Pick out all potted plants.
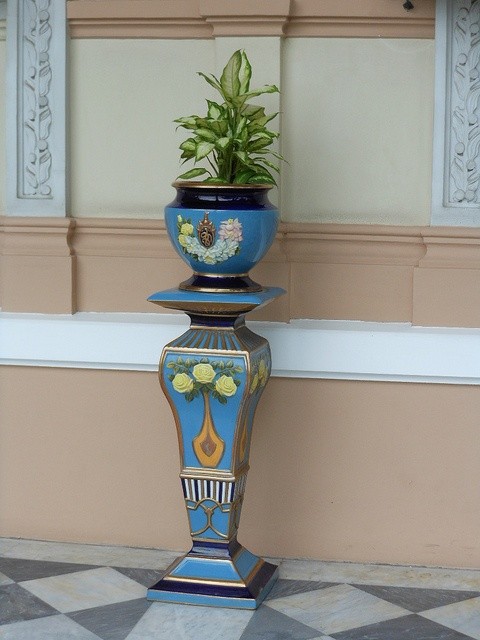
[166,50,288,294]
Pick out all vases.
[146,291,279,609]
